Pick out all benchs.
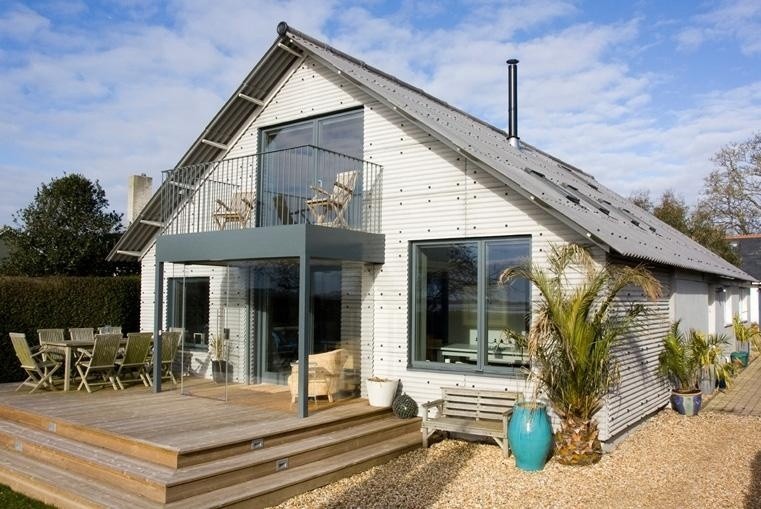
[421,386,528,458]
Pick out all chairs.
[10,322,182,396]
[306,169,358,229]
[214,191,255,231]
[272,196,313,225]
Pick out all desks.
[441,341,531,364]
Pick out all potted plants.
[730,316,761,370]
[208,335,232,384]
[656,318,742,417]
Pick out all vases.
[508,399,552,470]
[367,376,401,408]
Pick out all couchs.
[286,347,349,403]
